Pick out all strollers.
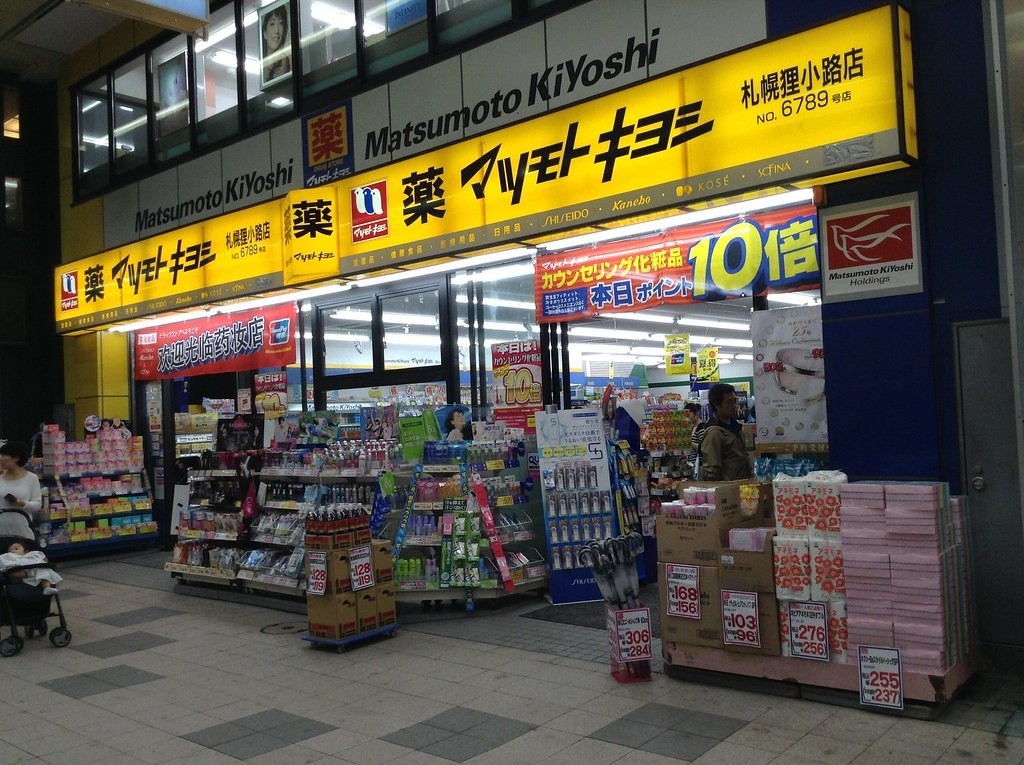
[0,509,72,657]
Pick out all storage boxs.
[656,479,976,674]
[303,519,397,640]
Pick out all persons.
[446,409,465,442]
[362,408,380,438]
[8,543,58,595]
[606,399,614,419]
[685,403,706,467]
[0,441,42,541]
[276,417,336,439]
[701,384,752,482]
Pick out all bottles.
[252,440,395,533]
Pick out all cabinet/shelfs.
[34,468,160,560]
[163,456,548,614]
